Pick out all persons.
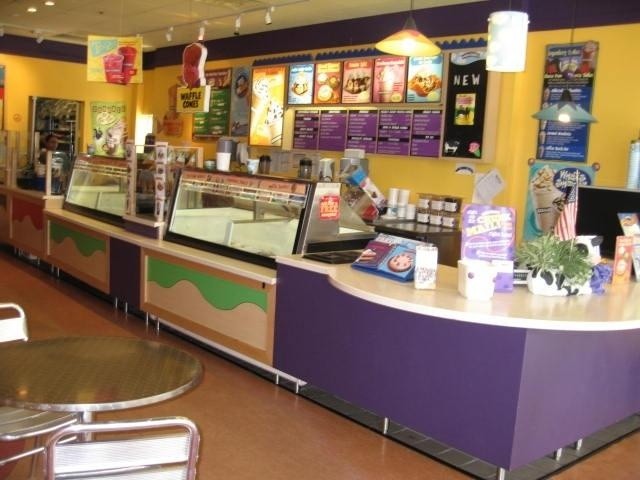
[38,134,59,165]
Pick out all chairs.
[42,415,202,480]
[0,302,83,479]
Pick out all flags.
[553,178,579,246]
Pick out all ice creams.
[529,165,557,231]
[250,77,284,146]
[467,141,481,157]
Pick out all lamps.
[483,2,531,76]
[0,25,45,44]
[162,5,273,44]
[531,1,597,128]
[373,0,442,60]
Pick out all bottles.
[298,159,312,179]
[416,191,462,228]
[259,155,271,174]
[627,139,640,190]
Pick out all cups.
[414,246,439,290]
[104,46,138,79]
[387,188,417,220]
[246,159,260,174]
[531,189,562,232]
[205,160,214,171]
[215,152,232,171]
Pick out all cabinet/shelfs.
[366,215,464,269]
[164,163,377,272]
[61,153,177,246]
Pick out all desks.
[0,335,207,443]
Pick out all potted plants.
[516,233,593,299]
